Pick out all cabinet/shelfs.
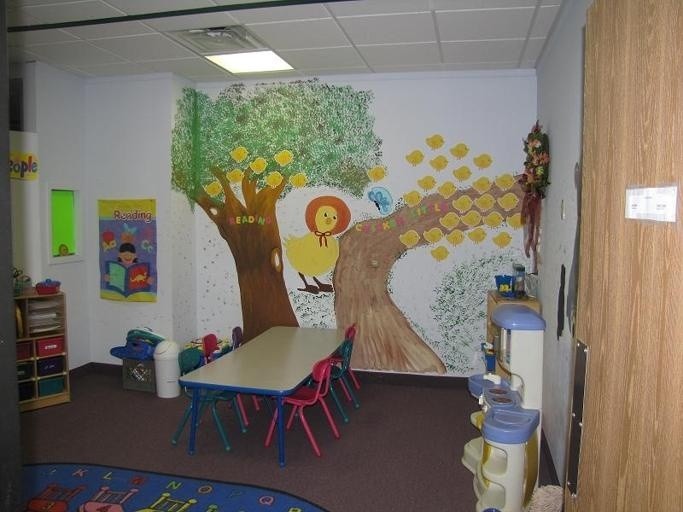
[12,285,71,413]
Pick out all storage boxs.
[16,335,65,402]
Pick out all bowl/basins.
[36,282,62,295]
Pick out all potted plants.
[11,265,31,296]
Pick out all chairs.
[171,322,361,468]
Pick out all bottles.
[46,279,52,287]
[514,264,525,291]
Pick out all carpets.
[20,463,328,512]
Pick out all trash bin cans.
[152,341,182,399]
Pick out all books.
[104,260,150,297]
[27,298,64,336]
